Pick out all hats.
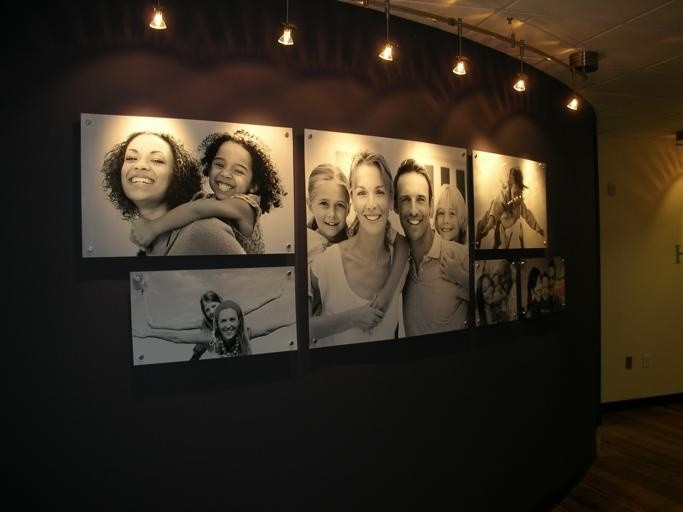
[508,168,529,189]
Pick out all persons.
[475,261,518,326]
[100,131,285,257]
[132,300,296,358]
[147,291,285,329]
[526,262,563,317]
[307,151,470,348]
[476,167,545,250]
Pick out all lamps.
[149,0,167,30]
[512,41,526,92]
[452,19,467,76]
[566,66,580,111]
[379,3,394,62]
[278,1,295,46]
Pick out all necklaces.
[218,336,239,356]
